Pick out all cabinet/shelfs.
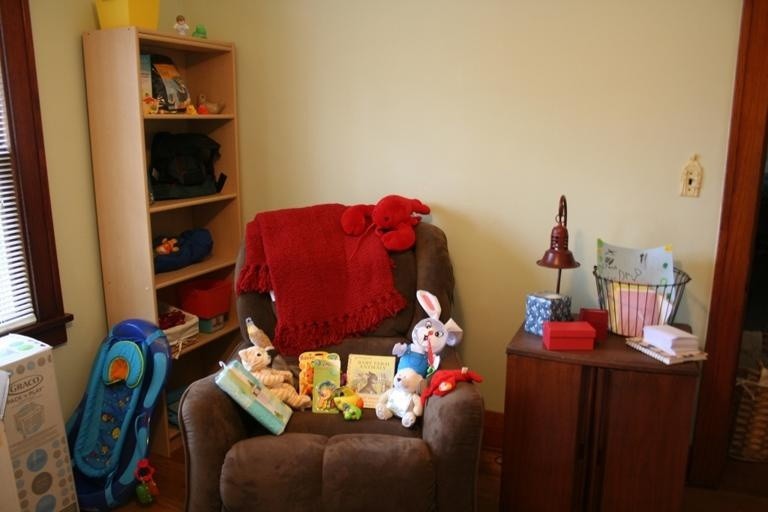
[500,323,700,511]
[82,27,245,457]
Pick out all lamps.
[536,194,579,294]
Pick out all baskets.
[591,267,691,337]
[159,300,199,355]
[728,364,767,465]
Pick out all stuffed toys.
[375,364,424,430]
[392,290,464,375]
[156,237,179,255]
[239,344,311,411]
[339,192,433,252]
[244,314,301,394]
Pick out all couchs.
[176,204,485,512]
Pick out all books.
[295,350,398,421]
[626,332,709,366]
